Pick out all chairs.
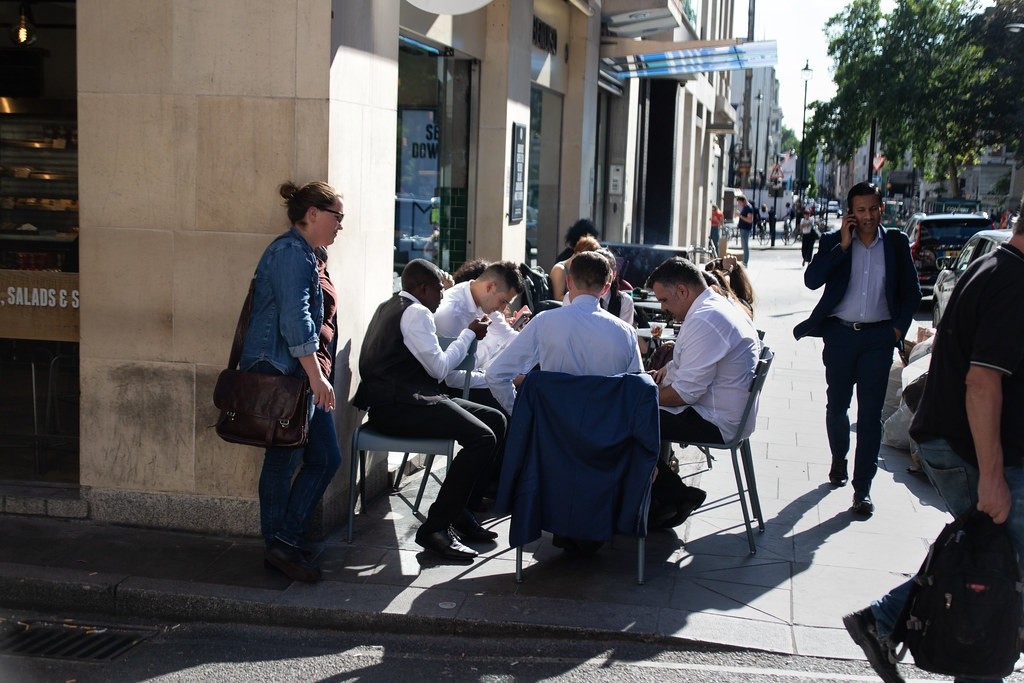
[347,264,775,585]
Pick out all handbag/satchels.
[213,368,309,447]
[812,228,821,240]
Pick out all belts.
[833,316,891,330]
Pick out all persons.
[237,180,343,584]
[842,190,1024,683]
[793,180,921,513]
[703,203,815,269]
[736,195,753,268]
[651,255,760,529]
[485,250,645,556]
[352,257,507,558]
[430,216,758,499]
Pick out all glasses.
[319,206,344,222]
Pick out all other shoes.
[676,485,705,516]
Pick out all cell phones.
[848,210,854,235]
[512,310,532,330]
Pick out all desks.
[601,241,689,284]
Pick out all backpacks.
[911,507,1024,674]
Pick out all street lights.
[752,89,765,240]
[796,58,814,225]
[769,152,786,248]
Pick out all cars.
[881,200,904,228]
[793,195,839,216]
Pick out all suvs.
[931,228,1015,331]
[902,209,998,310]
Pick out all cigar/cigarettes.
[328,402,336,413]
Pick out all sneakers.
[457,513,497,541]
[829,457,849,487]
[852,493,874,514]
[844,608,906,683]
[264,541,322,583]
[415,519,480,560]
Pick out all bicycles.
[781,217,797,246]
[756,219,771,246]
[722,218,731,241]
